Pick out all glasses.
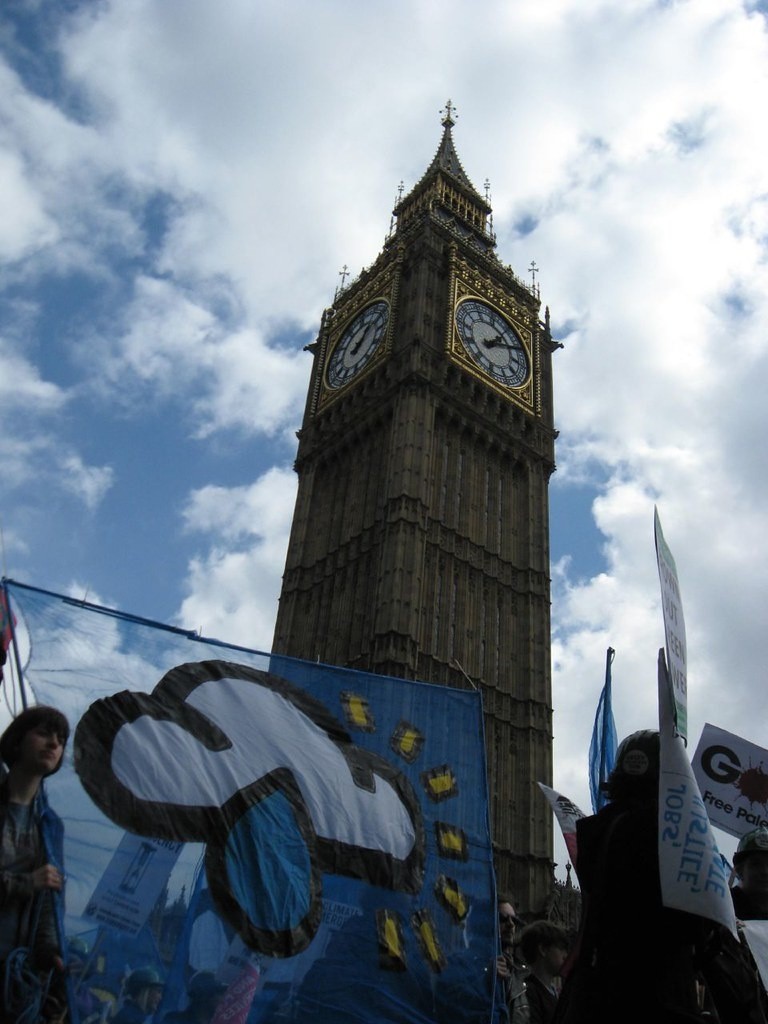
[498,912,520,925]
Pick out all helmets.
[732,825,768,875]
[608,729,660,797]
[67,938,87,955]
[121,966,167,996]
[185,969,228,998]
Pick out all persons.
[67,935,230,1024]
[498,730,768,1024]
[0,705,71,1024]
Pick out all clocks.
[322,297,391,391]
[453,296,532,390]
